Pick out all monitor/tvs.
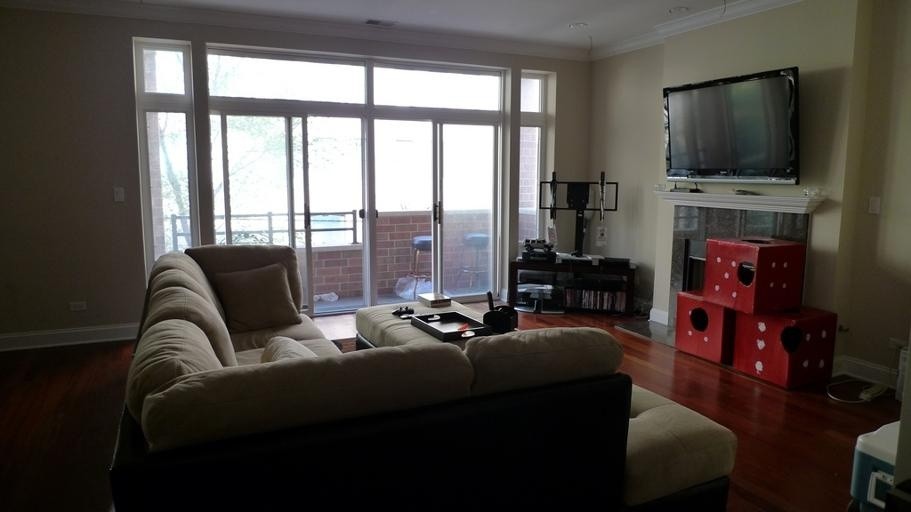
[663,66,800,184]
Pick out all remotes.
[399,313,424,319]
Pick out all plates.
[411,310,493,343]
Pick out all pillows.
[261,336,319,363]
[216,262,302,332]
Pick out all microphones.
[550,171,558,219]
[599,172,606,220]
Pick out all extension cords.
[859,383,890,403]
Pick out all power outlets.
[887,337,908,351]
[69,301,87,311]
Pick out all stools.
[408,236,432,301]
[454,233,490,290]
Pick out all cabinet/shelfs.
[509,262,634,316]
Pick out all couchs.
[110,245,739,512]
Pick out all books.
[417,292,451,307]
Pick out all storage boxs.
[851,420,901,509]
